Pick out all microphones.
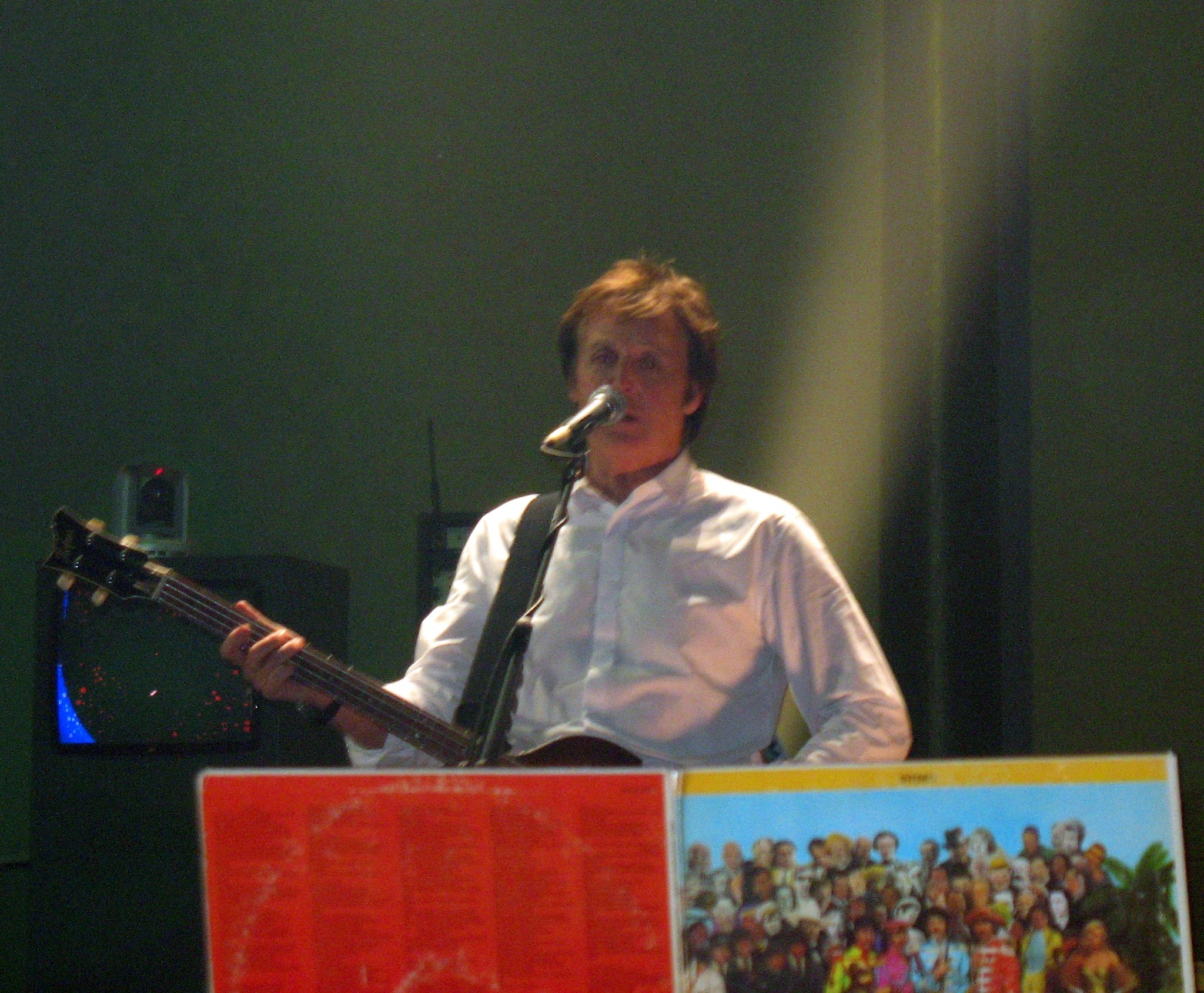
[540,384,627,451]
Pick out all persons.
[682,819,1139,993]
[222,258,911,770]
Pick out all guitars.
[37,501,658,768]
